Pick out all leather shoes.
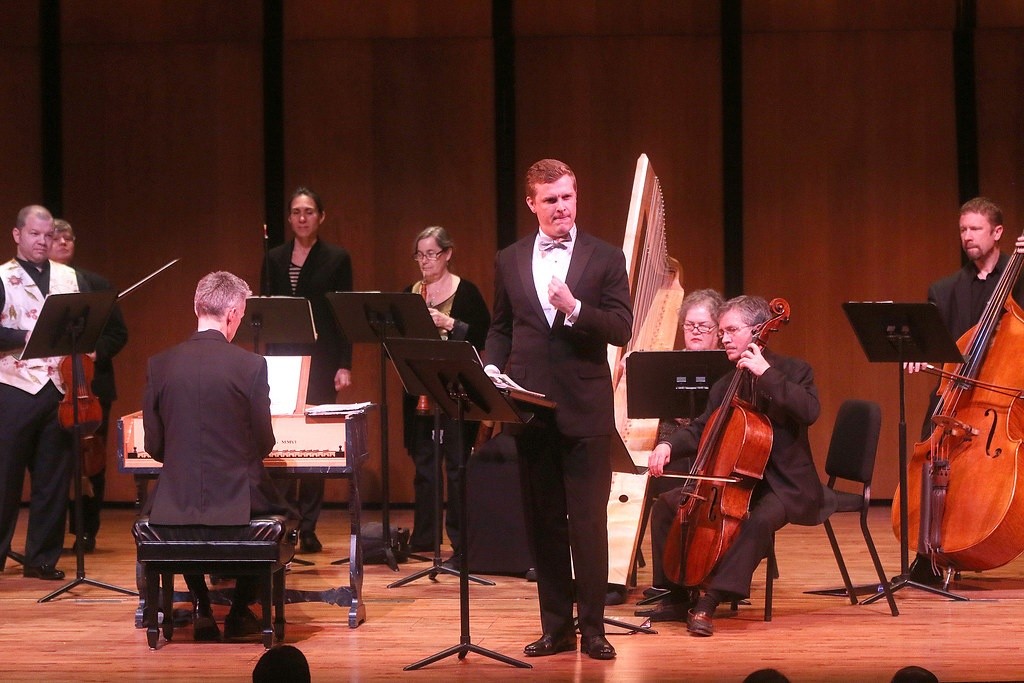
[581,634,618,660]
[634,598,691,622]
[192,599,220,639]
[524,634,577,656]
[739,600,751,605]
[224,613,276,636]
[73,533,95,553]
[23,564,65,578]
[687,608,713,635]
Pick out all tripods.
[842,301,970,605]
[626,349,753,608]
[326,290,536,672]
[20,289,140,604]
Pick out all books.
[490,371,545,398]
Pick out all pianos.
[116,402,375,630]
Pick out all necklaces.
[427,277,446,306]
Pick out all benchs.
[131,514,301,653]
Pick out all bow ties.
[538,231,572,251]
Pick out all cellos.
[890,250,1023,592]
[661,297,793,588]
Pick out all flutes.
[417,271,430,411]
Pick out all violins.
[57,354,104,441]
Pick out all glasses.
[681,320,718,333]
[412,249,444,262]
[53,234,76,245]
[716,322,762,338]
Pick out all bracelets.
[659,442,672,448]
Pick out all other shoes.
[440,555,460,574]
[299,533,323,552]
[406,542,436,553]
[891,563,944,586]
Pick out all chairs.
[731,399,899,623]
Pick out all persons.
[0,205,97,580]
[260,186,354,551]
[903,197,1024,584]
[891,666,939,683]
[387,227,491,571]
[50,219,130,553]
[253,645,311,683]
[482,157,633,660]
[643,287,836,635]
[141,271,302,641]
[743,669,789,683]
